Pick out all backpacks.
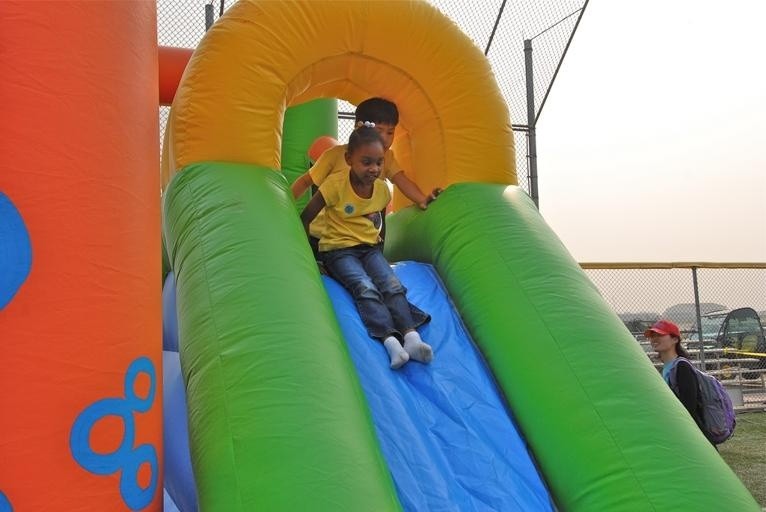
[669,357,736,444]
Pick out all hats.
[644,320,680,337]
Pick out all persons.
[291,97,444,263]
[644,321,720,453]
[300,126,433,370]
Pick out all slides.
[162,162,762,512]
